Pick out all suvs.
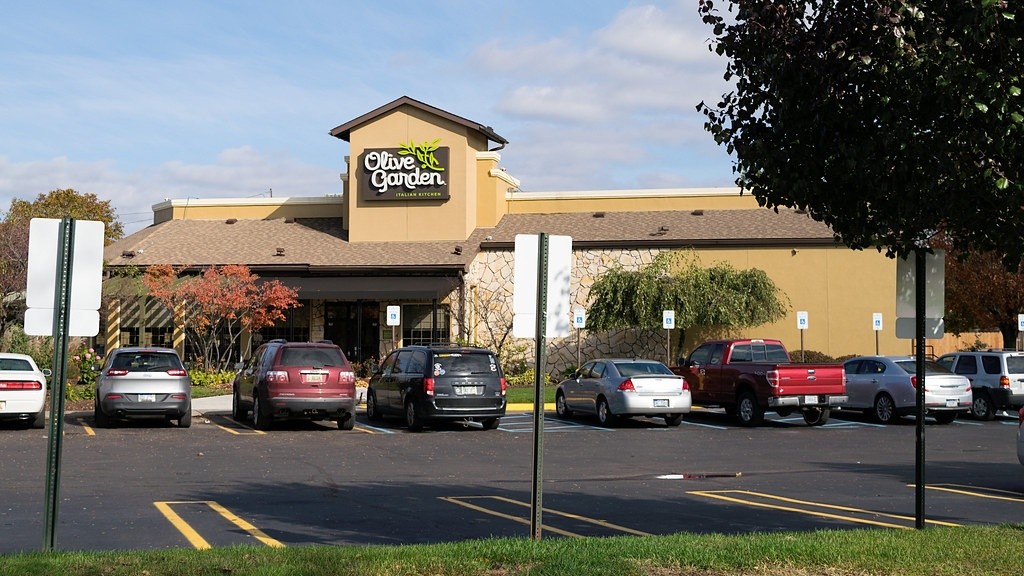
[932,350,1024,419]
[91,348,194,429]
[363,344,506,429]
[229,337,358,431]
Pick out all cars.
[553,355,695,427]
[0,351,53,428]
[826,355,974,429]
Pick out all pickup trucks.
[665,337,850,428]
